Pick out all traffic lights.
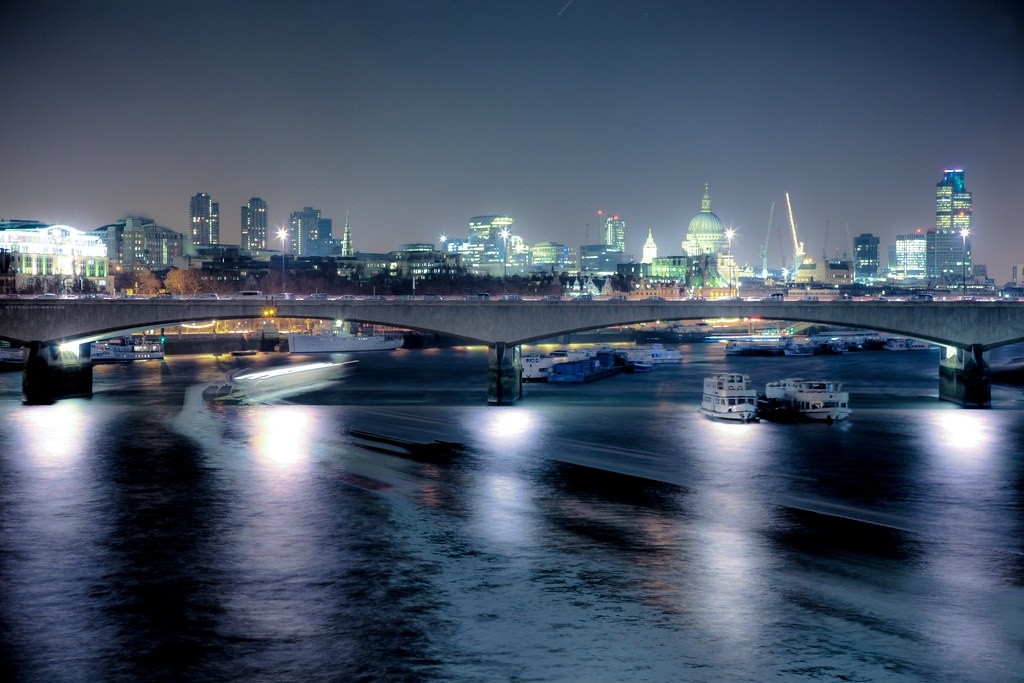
[161,335,165,344]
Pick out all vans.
[229,290,262,298]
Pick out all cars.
[541,292,851,301]
[271,293,524,301]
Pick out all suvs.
[200,294,219,301]
[906,293,933,303]
[149,291,174,300]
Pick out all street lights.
[279,228,287,291]
[726,230,733,299]
[501,229,508,292]
[961,228,968,293]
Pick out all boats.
[520,342,683,384]
[698,371,757,423]
[86,336,165,361]
[724,340,849,357]
[283,328,405,354]
[702,339,721,343]
[1,347,25,369]
[884,336,929,351]
[632,323,714,345]
[756,376,852,422]
[234,350,255,355]
